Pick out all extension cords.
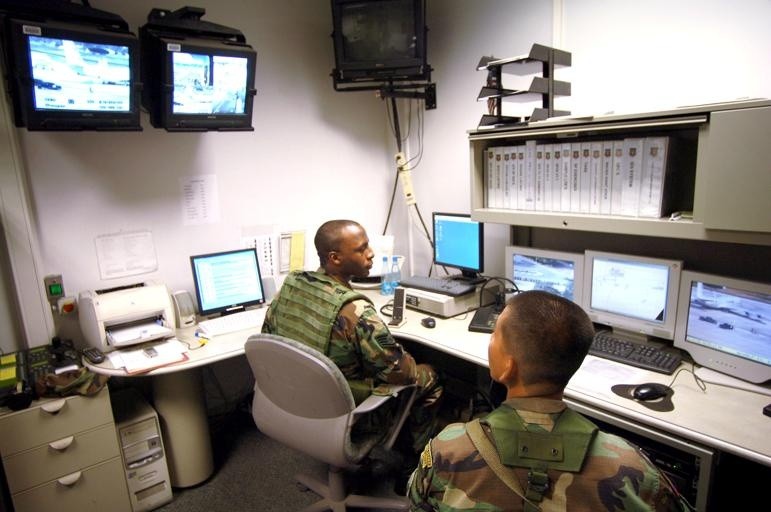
[394,151,416,205]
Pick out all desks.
[76,284,771,510]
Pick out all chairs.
[245,332,418,512]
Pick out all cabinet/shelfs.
[476,44,572,128]
[0,380,131,512]
[467,103,706,240]
[700,99,770,246]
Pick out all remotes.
[82,347,104,364]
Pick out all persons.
[255,217,448,495]
[405,287,698,512]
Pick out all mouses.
[634,383,673,401]
[422,318,436,329]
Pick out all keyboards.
[586,329,682,372]
[198,306,269,338]
[469,309,500,333]
[400,275,476,297]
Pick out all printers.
[78,272,176,354]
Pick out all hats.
[36,367,109,397]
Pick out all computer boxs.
[111,386,174,512]
[396,285,500,317]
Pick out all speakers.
[172,289,197,329]
[262,276,277,304]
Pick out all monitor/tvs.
[332,0,426,80]
[674,268,771,384]
[140,7,256,132]
[504,255,582,304]
[0,0,140,131]
[433,212,487,285]
[585,249,682,347]
[190,248,266,316]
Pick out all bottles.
[391,256,401,289]
[382,256,391,295]
